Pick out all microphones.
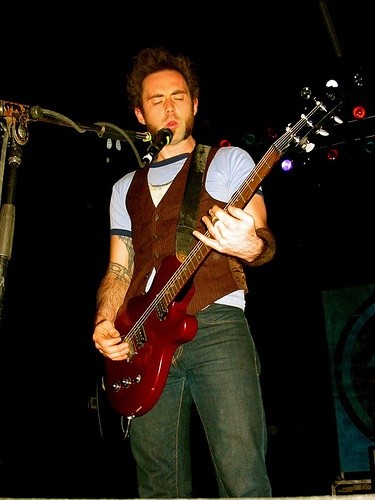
[140,127,174,168]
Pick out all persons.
[90,54,277,500]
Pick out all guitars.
[102,84,344,440]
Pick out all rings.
[212,218,220,227]
[102,352,108,356]
